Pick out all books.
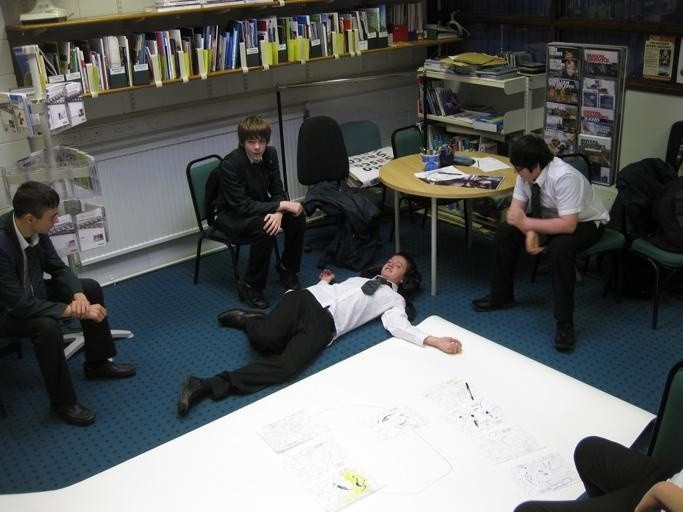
[12,2,682,98]
[418,99,622,221]
[344,144,395,190]
[0,81,111,255]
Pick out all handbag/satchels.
[204,166,218,227]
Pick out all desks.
[378,151,519,296]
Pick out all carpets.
[1,202,683,494]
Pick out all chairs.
[391,125,468,234]
[0,316,73,416]
[630,120,683,330]
[530,152,625,282]
[297,115,389,271]
[186,154,280,303]
[645,360,683,479]
[340,120,387,248]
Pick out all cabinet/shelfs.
[416,69,546,239]
[426,0,683,97]
[4,0,465,122]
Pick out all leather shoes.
[274,260,301,291]
[82,359,136,379]
[176,374,202,418]
[553,314,576,352]
[49,401,96,426]
[235,279,271,310]
[217,308,267,332]
[470,285,516,311]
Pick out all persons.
[204,115,307,308]
[473,134,610,350]
[0,181,136,427]
[176,250,461,415]
[513,436,682,511]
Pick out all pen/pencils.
[418,145,441,155]
[477,161,479,167]
[466,382,474,400]
[437,172,462,175]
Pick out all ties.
[530,183,541,218]
[24,245,49,301]
[360,275,393,296]
